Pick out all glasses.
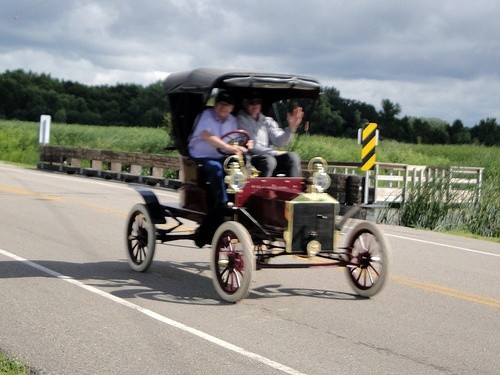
[248,100,263,104]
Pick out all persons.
[187,91,254,210]
[234,92,306,179]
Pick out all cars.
[125,66,389,302]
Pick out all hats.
[215,90,236,104]
